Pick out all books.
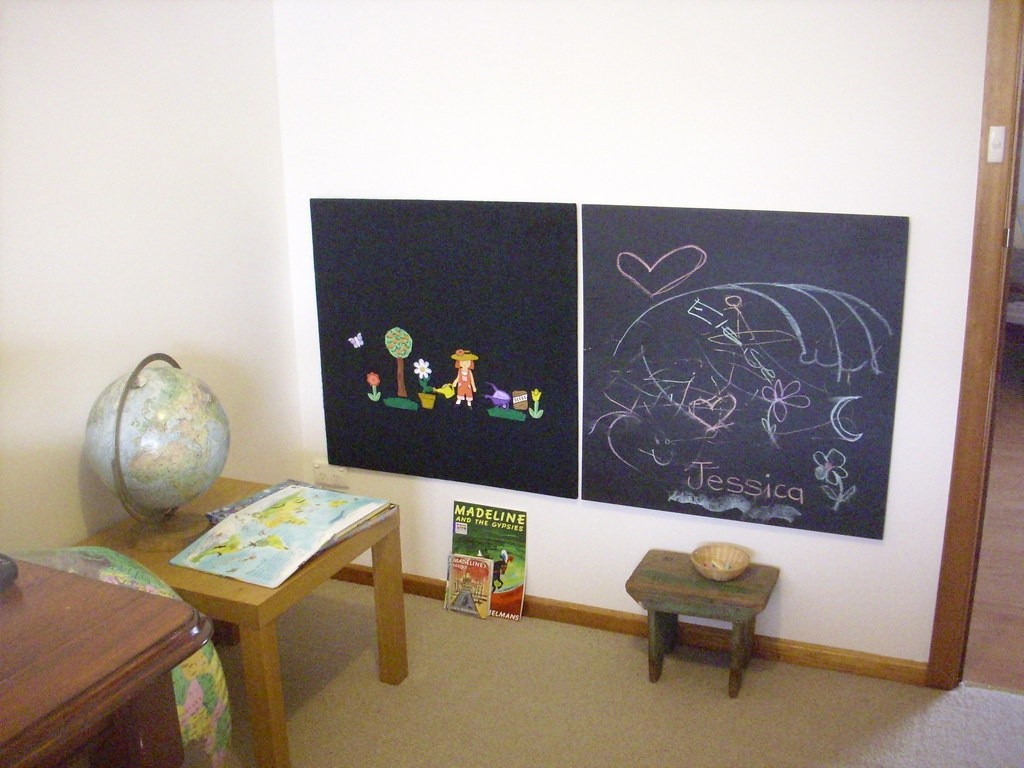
[168,475,395,588]
[442,499,528,621]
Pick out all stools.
[624,549,782,699]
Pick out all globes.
[1,545,231,767]
[85,352,230,553]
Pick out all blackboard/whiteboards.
[310,197,579,498]
[580,202,909,540]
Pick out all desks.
[71,470,410,768]
[1,552,216,768]
